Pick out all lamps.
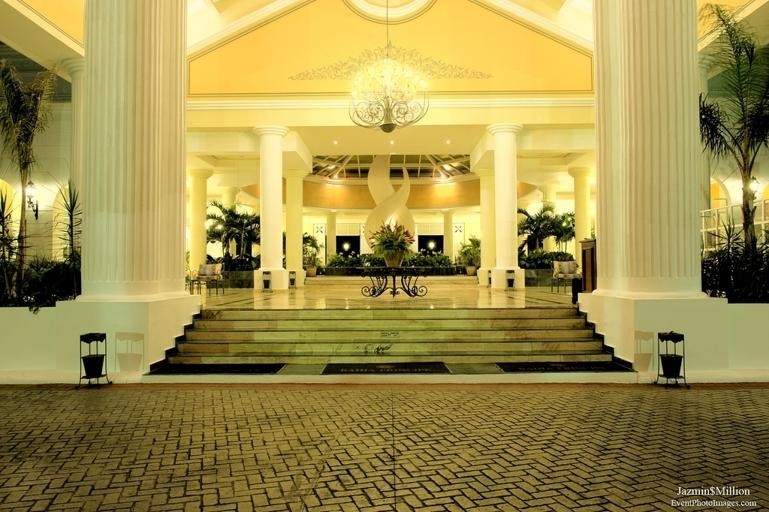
[348,1,430,133]
[26,180,39,220]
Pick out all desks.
[360,266,428,298]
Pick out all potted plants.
[303,248,322,277]
[458,235,481,276]
[368,217,419,267]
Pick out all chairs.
[550,260,581,294]
[190,263,226,295]
